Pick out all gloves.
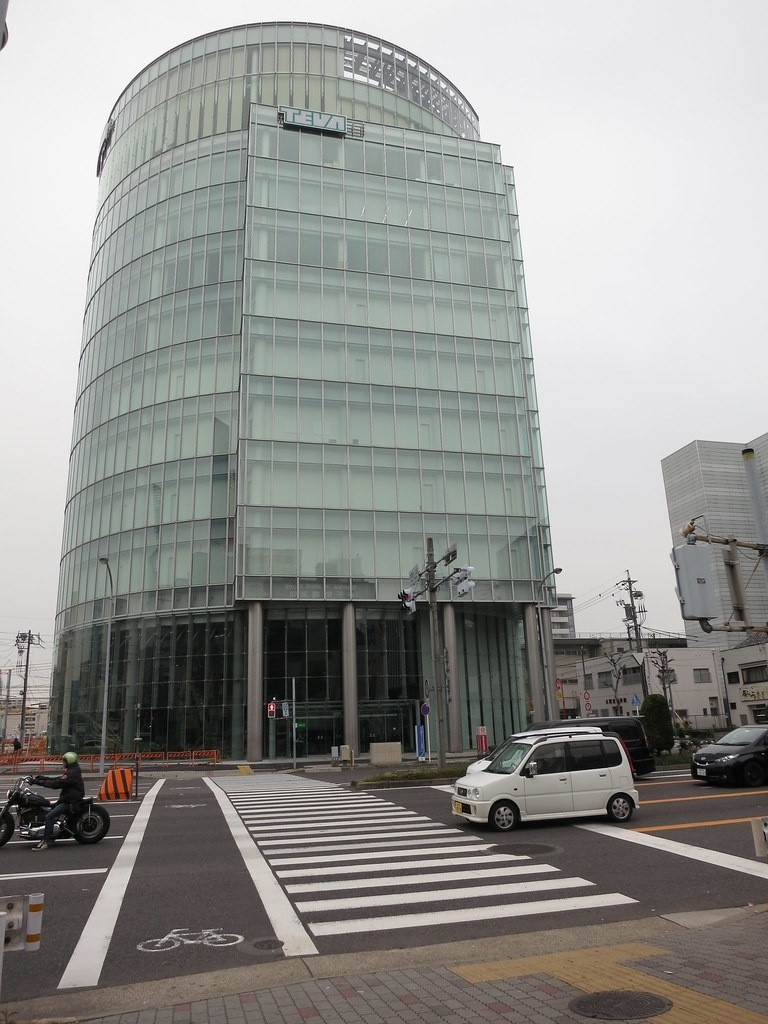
[35,775,49,780]
[29,779,44,786]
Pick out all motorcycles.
[0,774,111,847]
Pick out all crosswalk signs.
[631,695,641,707]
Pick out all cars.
[691,725,768,785]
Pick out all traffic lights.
[268,703,276,719]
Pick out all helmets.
[62,752,78,769]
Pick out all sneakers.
[32,840,48,852]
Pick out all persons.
[28,752,85,851]
[13,737,21,751]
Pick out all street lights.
[99,557,113,774]
[537,568,565,720]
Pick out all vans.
[522,716,654,777]
[450,733,639,830]
[466,726,602,776]
